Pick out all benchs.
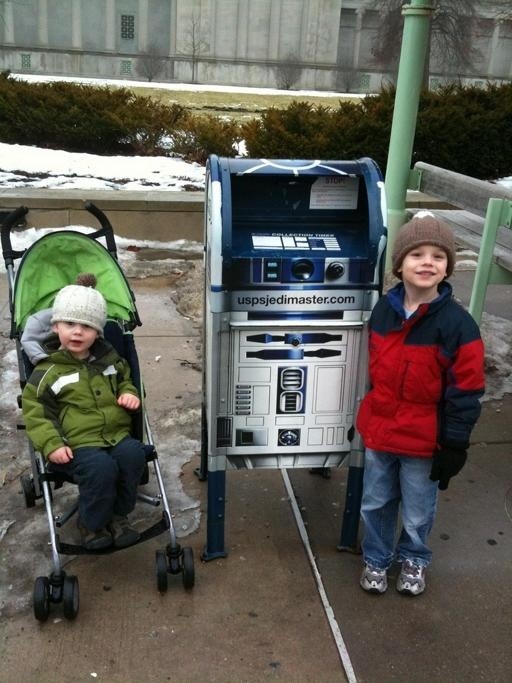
[413,162,511,327]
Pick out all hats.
[48,284,107,337]
[391,216,456,281]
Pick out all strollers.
[1,202,195,623]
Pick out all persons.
[22,271,148,551]
[356,208,485,597]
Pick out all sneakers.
[75,512,142,553]
[357,555,427,598]
